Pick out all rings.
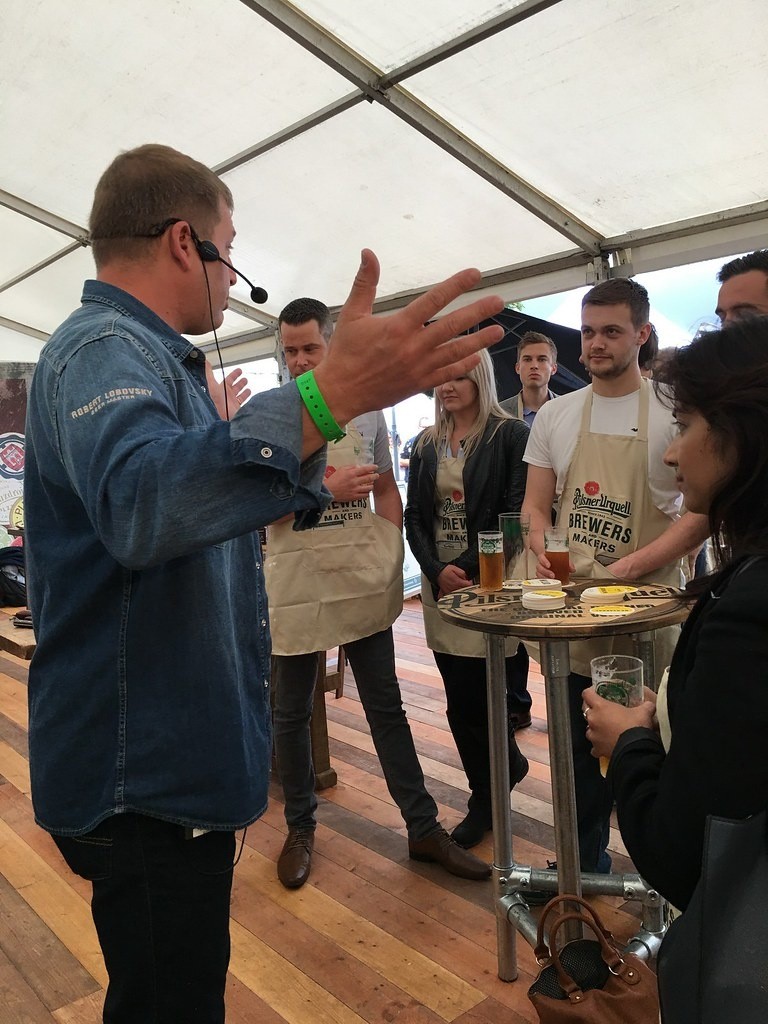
[583,708,591,719]
[585,725,590,731]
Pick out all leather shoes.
[408,829,494,880]
[277,826,314,890]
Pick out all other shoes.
[580,844,612,877]
[507,711,532,729]
[509,756,530,796]
[520,862,557,911]
[449,799,489,849]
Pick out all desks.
[0,603,342,789]
[437,576,697,983]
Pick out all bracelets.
[295,369,347,446]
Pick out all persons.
[638,320,711,580]
[404,337,533,850]
[497,330,563,726]
[714,249,768,328]
[580,313,768,914]
[519,277,715,906]
[25,145,503,1024]
[259,298,492,891]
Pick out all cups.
[498,512,530,586]
[478,531,504,592]
[590,655,644,777]
[544,526,569,585]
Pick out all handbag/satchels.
[527,894,660,1024]
